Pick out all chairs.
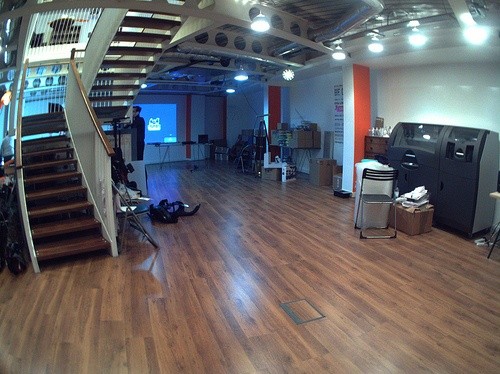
[111,178,159,252]
[232,140,256,172]
[212,140,231,162]
[354,168,399,241]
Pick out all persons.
[0,130,14,164]
[124,105,149,188]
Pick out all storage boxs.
[309,158,337,185]
[261,167,279,179]
[290,131,321,147]
[282,165,298,181]
[389,202,434,234]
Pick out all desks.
[155,142,197,167]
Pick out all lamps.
[407,24,427,47]
[225,83,236,93]
[234,62,249,81]
[366,30,384,52]
[251,7,270,32]
[331,41,347,61]
[281,65,296,80]
